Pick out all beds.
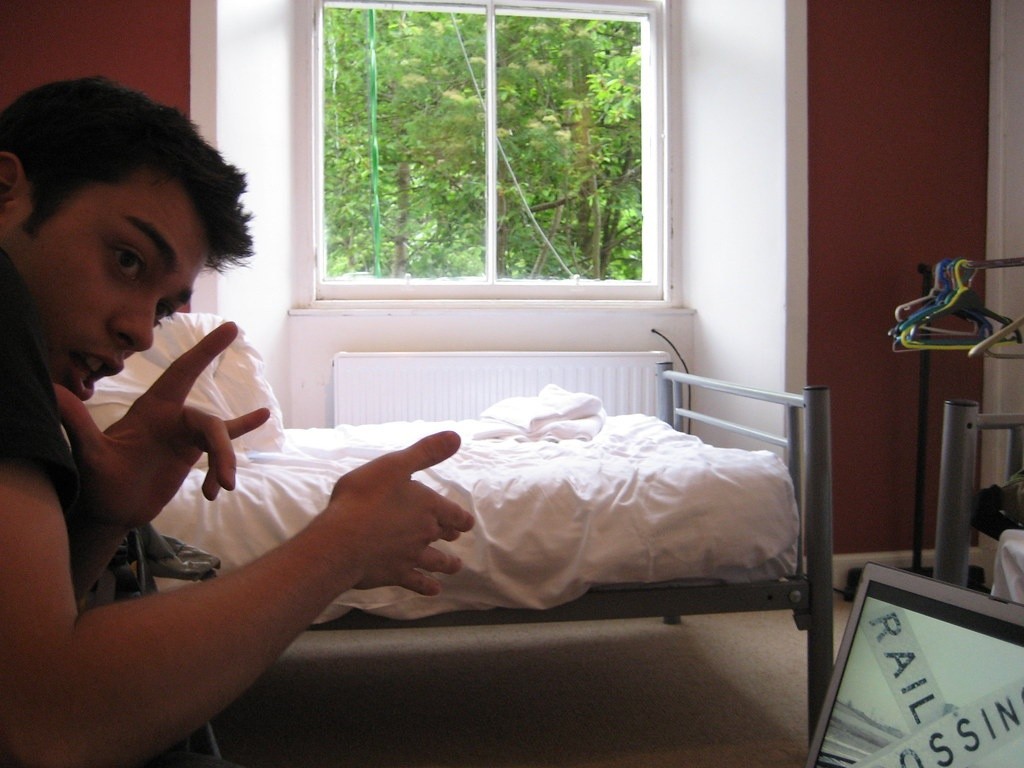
[81,311,834,753]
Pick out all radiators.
[331,351,672,428]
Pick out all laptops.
[804,561,1024,768]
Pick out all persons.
[1,71,476,768]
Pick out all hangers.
[888,257,1024,360]
